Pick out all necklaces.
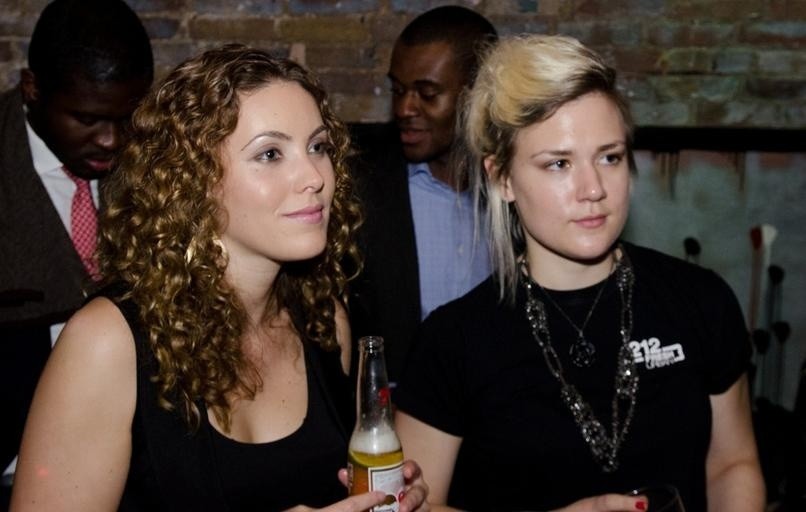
[530,254,619,368]
[516,246,638,475]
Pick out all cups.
[624,484,686,512]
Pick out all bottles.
[348,336,404,512]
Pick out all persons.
[336,5,504,391]
[387,30,766,512]
[0,1,158,512]
[6,38,432,512]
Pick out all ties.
[60,165,104,283]
